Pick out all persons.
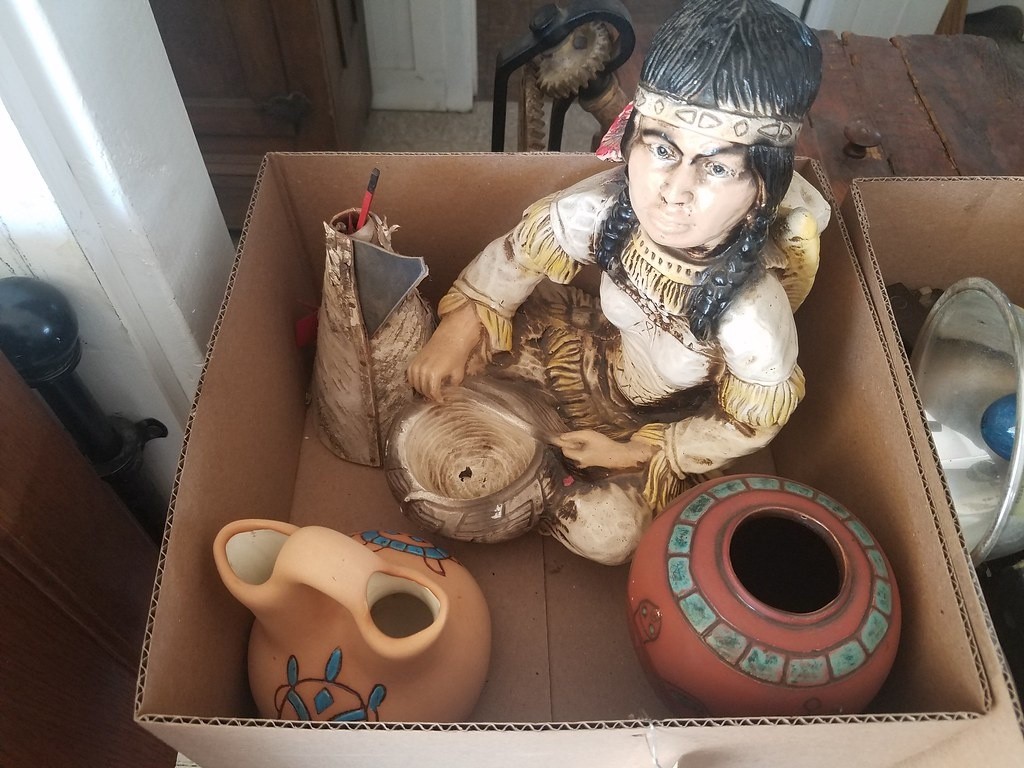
[408,0,827,565]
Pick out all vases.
[628,474,902,719]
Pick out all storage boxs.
[134,151,990,768]
[839,176,1024,768]
